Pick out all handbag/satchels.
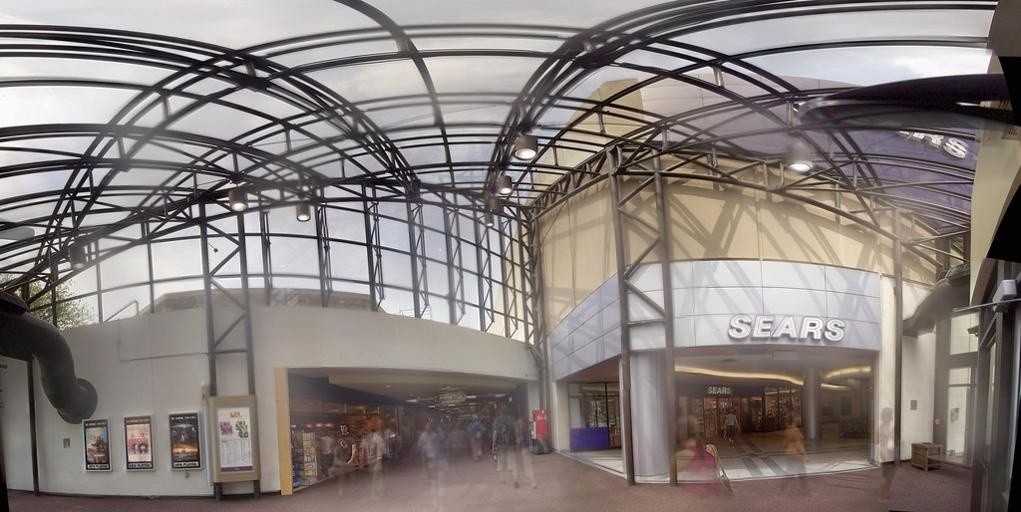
[709,445,734,499]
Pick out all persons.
[875,405,901,505]
[671,414,729,500]
[722,407,743,449]
[773,411,812,495]
[139,439,149,460]
[318,403,539,511]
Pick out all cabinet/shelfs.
[910,443,943,473]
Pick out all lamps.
[65,229,91,271]
[496,169,514,196]
[293,189,311,222]
[479,194,495,228]
[228,173,249,212]
[781,99,816,174]
[509,119,539,161]
